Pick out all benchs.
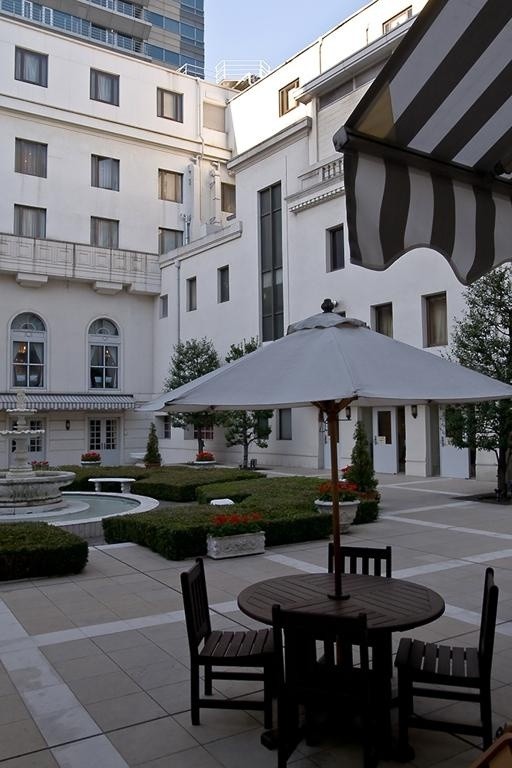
[88,478,136,494]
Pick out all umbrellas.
[132,296,511,596]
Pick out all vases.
[206,531,266,560]
[194,461,216,468]
[314,499,361,533]
[81,461,102,467]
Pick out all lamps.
[345,406,351,419]
[411,405,417,418]
[66,419,70,430]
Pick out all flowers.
[316,480,358,502]
[207,513,261,538]
[81,451,101,461]
[195,451,215,461]
